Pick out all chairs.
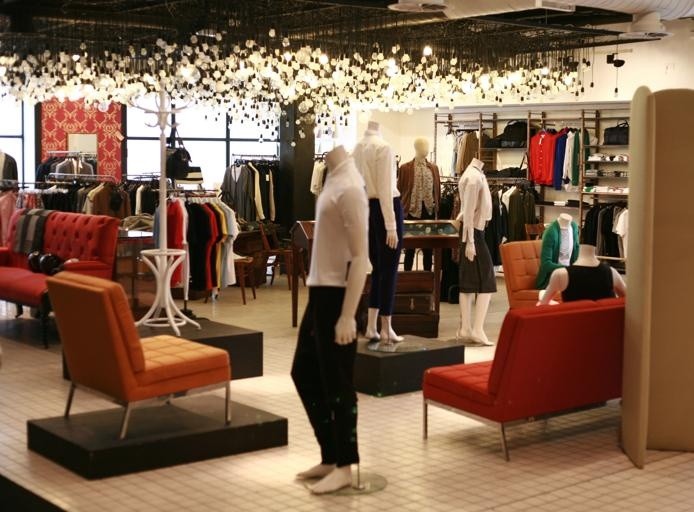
[524,223,545,239]
[500,239,565,303]
[205,256,257,304]
[259,220,305,289]
[45,270,231,440]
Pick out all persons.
[395,136,440,272]
[532,212,581,290]
[536,242,627,308]
[350,119,405,342]
[289,144,370,496]
[457,157,499,348]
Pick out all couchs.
[422,297,626,461]
[0,211,122,348]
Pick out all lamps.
[0,0,624,144]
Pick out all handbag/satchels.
[166,128,192,179]
[501,120,534,147]
[604,119,629,145]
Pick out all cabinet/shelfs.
[433,109,629,259]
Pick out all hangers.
[0,150,280,208]
[593,195,627,215]
[442,179,527,195]
